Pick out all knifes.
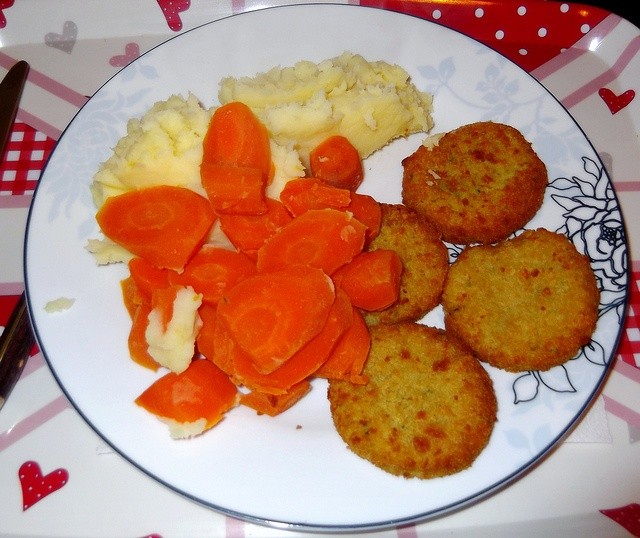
[0,60,29,169]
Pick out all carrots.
[96,103,403,439]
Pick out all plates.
[23,3,632,534]
